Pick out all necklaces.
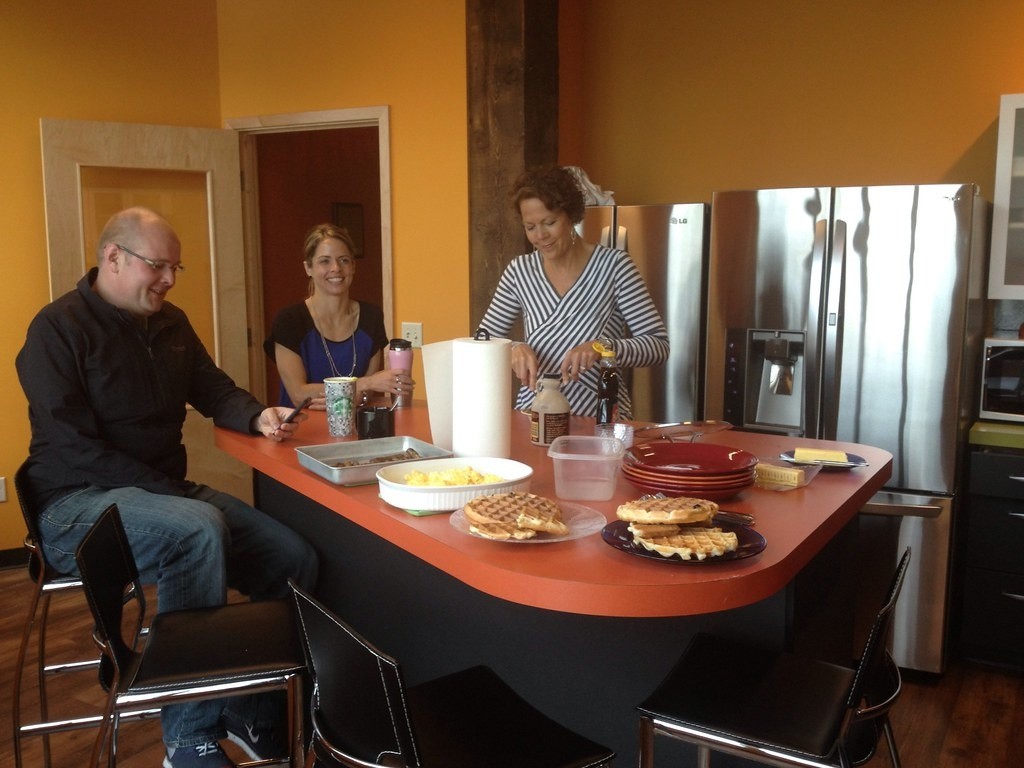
[312,301,357,377]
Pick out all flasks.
[980,337,1024,422]
[388,339,413,408]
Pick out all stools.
[285,578,619,767]
[75,502,306,768]
[637,545,912,768]
[11,457,162,768]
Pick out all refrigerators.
[572,184,994,676]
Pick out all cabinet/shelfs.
[960,446,1024,671]
[986,91,1024,305]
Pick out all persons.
[262,223,415,410]
[13,203,318,768]
[473,160,671,421]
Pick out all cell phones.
[272,396,312,434]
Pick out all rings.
[579,366,587,370]
[395,376,401,381]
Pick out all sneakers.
[162,740,238,768]
[221,718,292,768]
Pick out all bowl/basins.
[547,435,626,502]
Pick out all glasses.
[118,246,185,276]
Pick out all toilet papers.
[419,337,514,461]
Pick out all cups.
[323,376,358,437]
[355,405,395,439]
[594,423,634,475]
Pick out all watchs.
[359,390,368,407]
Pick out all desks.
[212,407,895,768]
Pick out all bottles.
[531,374,570,446]
[592,343,619,425]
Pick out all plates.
[376,457,535,510]
[621,442,758,502]
[600,519,768,565]
[449,502,607,543]
[780,450,869,473]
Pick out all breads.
[793,447,850,462]
[465,491,569,540]
[755,463,805,486]
[615,496,737,561]
